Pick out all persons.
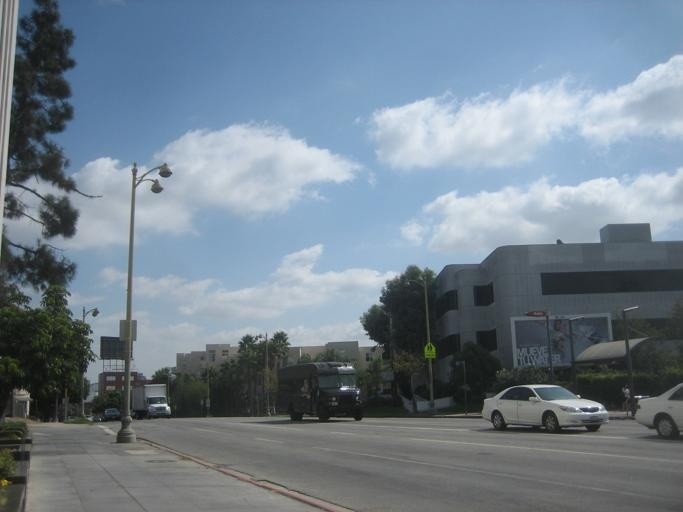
[621,384,632,411]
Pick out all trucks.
[132,382,172,419]
[282,361,362,422]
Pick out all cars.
[634,381,683,440]
[480,383,609,434]
[88,408,145,422]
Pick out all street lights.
[113,161,170,448]
[255,330,271,415]
[401,275,434,412]
[566,314,584,394]
[80,304,97,407]
[622,305,642,418]
